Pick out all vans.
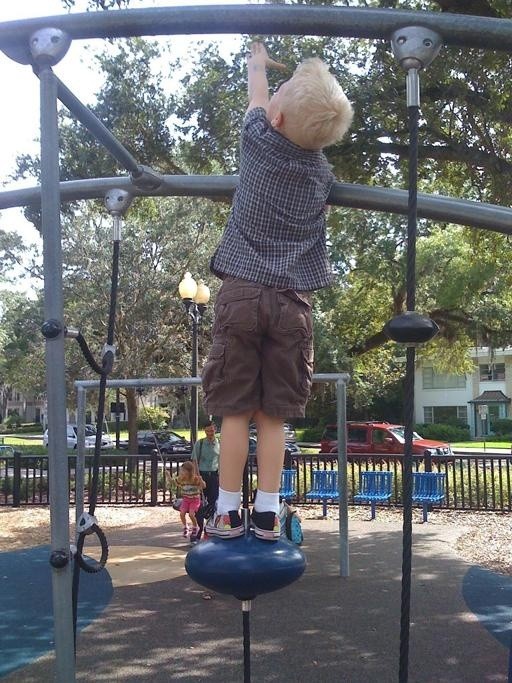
[43,423,113,449]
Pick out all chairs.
[411,472,445,522]
[305,470,339,517]
[353,471,393,518]
[279,468,297,506]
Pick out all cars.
[248,421,301,468]
[119,431,192,454]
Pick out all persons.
[190,419,222,539]
[165,461,206,538]
[200,40,354,540]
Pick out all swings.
[137,385,209,514]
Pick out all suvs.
[319,420,455,464]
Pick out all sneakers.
[182,523,201,537]
[247,504,281,542]
[202,508,245,539]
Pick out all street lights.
[178,272,210,444]
